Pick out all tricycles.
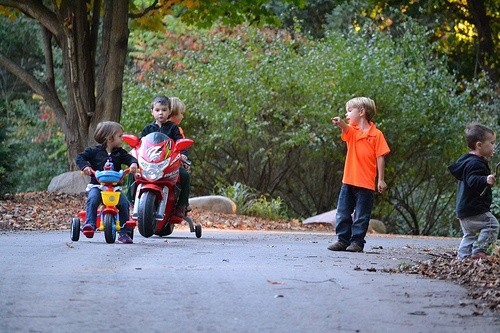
[69,161,137,244]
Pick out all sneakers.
[346,243,363,252]
[82,224,94,238]
[327,240,348,250]
[116,232,133,243]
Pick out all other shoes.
[185,204,191,212]
[472,252,492,259]
[177,206,185,217]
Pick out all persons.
[127,96,190,217]
[169,97,192,213]
[76,121,138,243]
[327,97,390,251]
[448,124,499,260]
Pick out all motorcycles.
[121,131,202,238]
